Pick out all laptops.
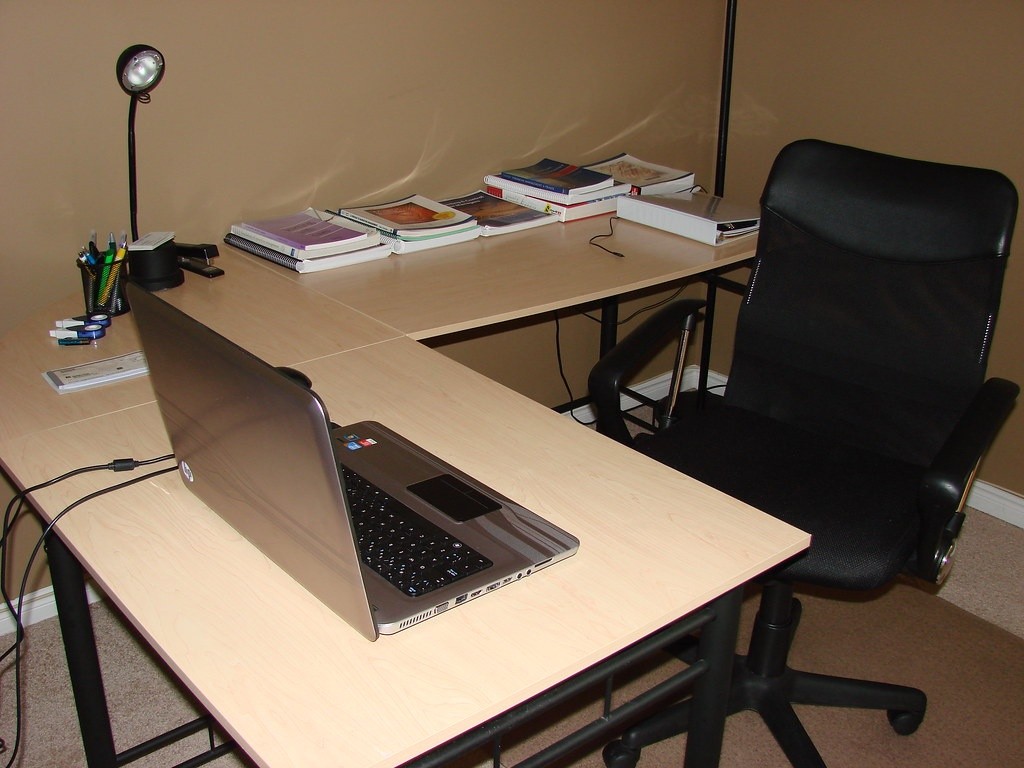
[123,280,579,642]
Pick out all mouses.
[275,366,312,391]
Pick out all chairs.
[589,137,1019,768]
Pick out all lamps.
[113,44,167,243]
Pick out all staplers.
[177,242,225,279]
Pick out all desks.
[0,192,813,768]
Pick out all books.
[583,152,696,196]
[617,192,761,247]
[338,193,481,254]
[484,158,632,222]
[223,207,391,274]
[437,190,559,237]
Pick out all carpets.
[482,585,1024,768]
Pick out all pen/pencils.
[77,230,128,313]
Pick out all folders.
[617,191,760,247]
